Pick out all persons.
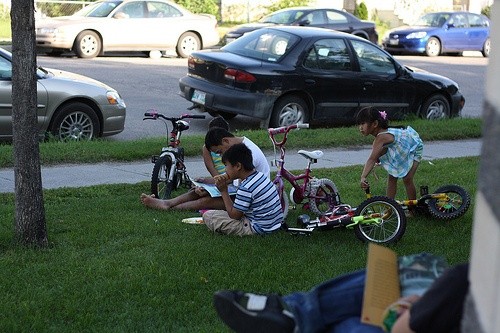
[203,143,284,236]
[356,107,424,217]
[214,254,470,333]
[140,127,270,210]
[202,115,229,176]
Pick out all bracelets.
[382,307,404,333]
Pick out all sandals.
[213,289,297,333]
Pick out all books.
[189,177,237,197]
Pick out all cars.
[0,48,127,144]
[221,6,379,58]
[178,27,467,133]
[35,0,220,59]
[382,10,492,57]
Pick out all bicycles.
[286,177,473,247]
[266,121,341,218]
[142,110,205,199]
